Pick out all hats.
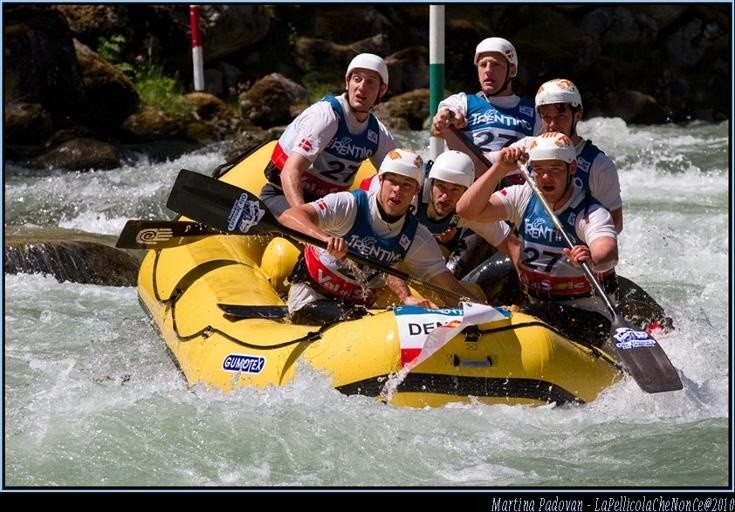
[377,150,426,186]
[536,79,580,107]
[527,132,576,163]
[473,37,517,64]
[345,53,388,83]
[428,150,475,188]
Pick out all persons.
[433,36,545,164]
[276,148,483,332]
[259,54,404,218]
[455,132,618,349]
[430,79,622,235]
[416,151,524,282]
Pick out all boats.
[137,139,669,411]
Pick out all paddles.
[444,110,675,334]
[166,169,482,304]
[115,220,281,250]
[516,160,683,393]
